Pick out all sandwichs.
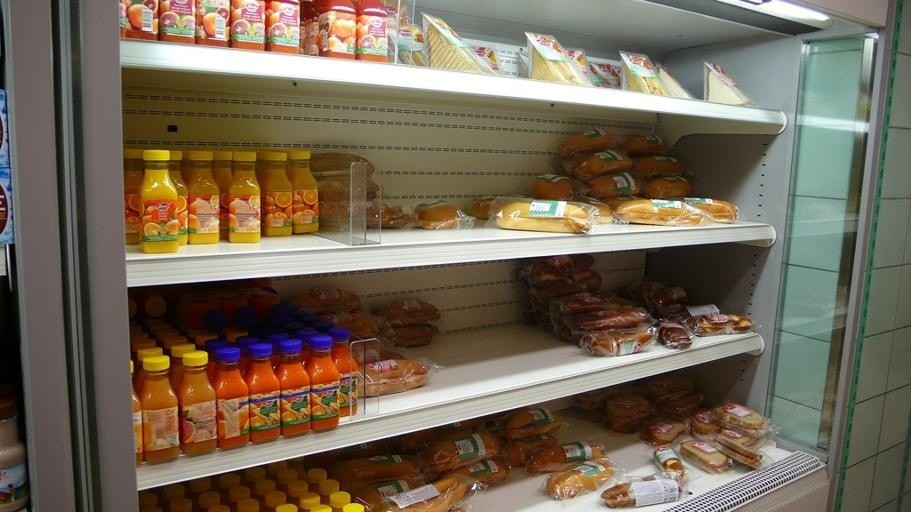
[390,11,754,106]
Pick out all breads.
[512,253,753,359]
[310,130,738,234]
[290,284,443,399]
[321,386,767,512]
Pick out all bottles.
[139,457,364,512]
[123,147,321,252]
[0,399,30,511]
[128,277,357,463]
[120,2,390,63]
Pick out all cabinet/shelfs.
[77,0,890,511]
